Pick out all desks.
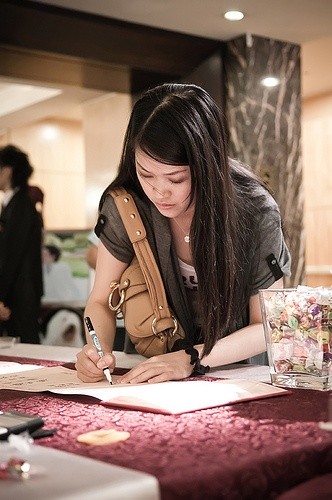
[0,344,332,500]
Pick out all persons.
[44,245,77,301]
[0,144,44,346]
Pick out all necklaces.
[173,217,192,244]
[74,84,292,385]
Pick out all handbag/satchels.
[107,185,186,359]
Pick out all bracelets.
[184,343,211,379]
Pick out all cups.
[259,289,332,392]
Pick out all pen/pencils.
[84,316,113,385]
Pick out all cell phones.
[0,411,44,440]
